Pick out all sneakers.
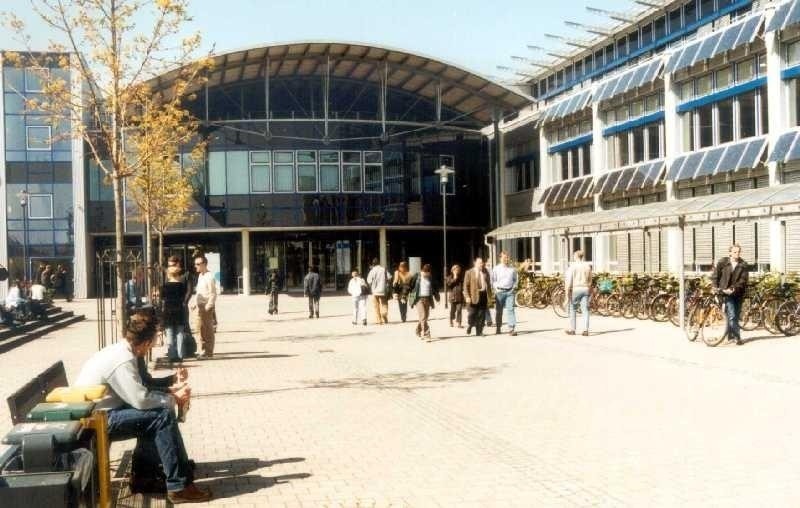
[129,478,165,493]
[168,483,209,503]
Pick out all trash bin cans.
[0,384,110,508]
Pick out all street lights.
[64,207,73,243]
[432,164,456,309]
[15,189,32,296]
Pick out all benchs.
[7,360,117,508]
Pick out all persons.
[125,247,218,363]
[347,271,368,326]
[564,250,593,336]
[367,258,391,324]
[127,311,198,494]
[711,242,750,347]
[448,252,519,337]
[265,272,281,315]
[410,264,440,342]
[304,267,321,318]
[1,263,68,328]
[70,312,212,503]
[393,261,413,322]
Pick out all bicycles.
[686,292,735,347]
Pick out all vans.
[14,256,115,295]
